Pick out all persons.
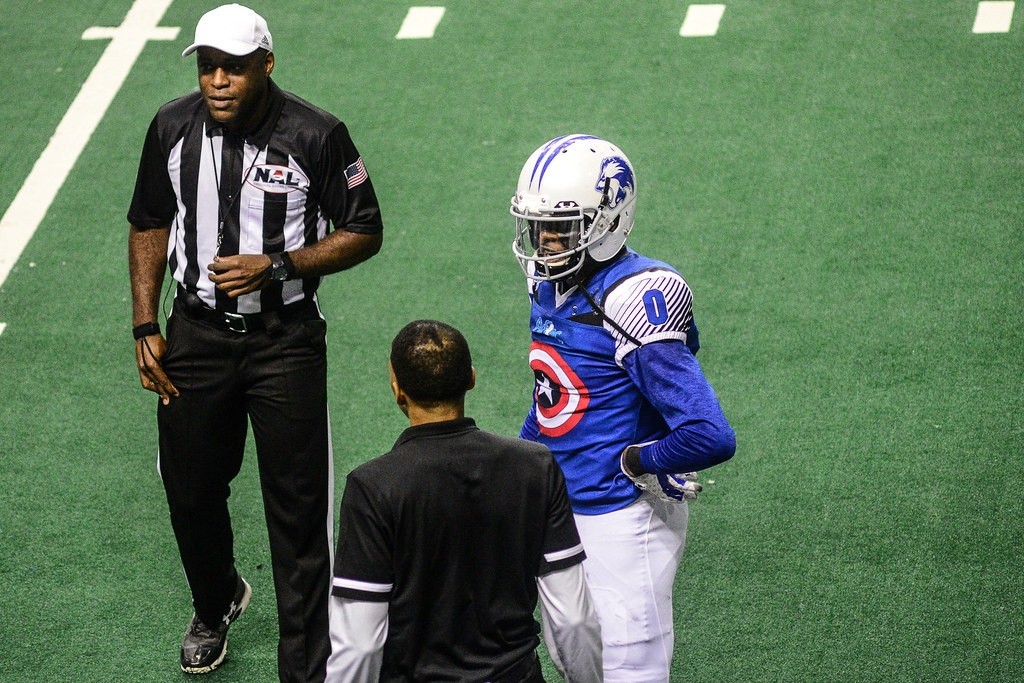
[510,134,737,683]
[126,5,384,683]
[324,320,604,683]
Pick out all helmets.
[509,133,636,282]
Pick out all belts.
[177,281,313,338]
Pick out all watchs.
[268,252,291,284]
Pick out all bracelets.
[132,322,161,340]
[279,251,297,277]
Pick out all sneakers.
[180,576,252,674]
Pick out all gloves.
[620,440,703,504]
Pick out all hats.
[181,3,273,56]
